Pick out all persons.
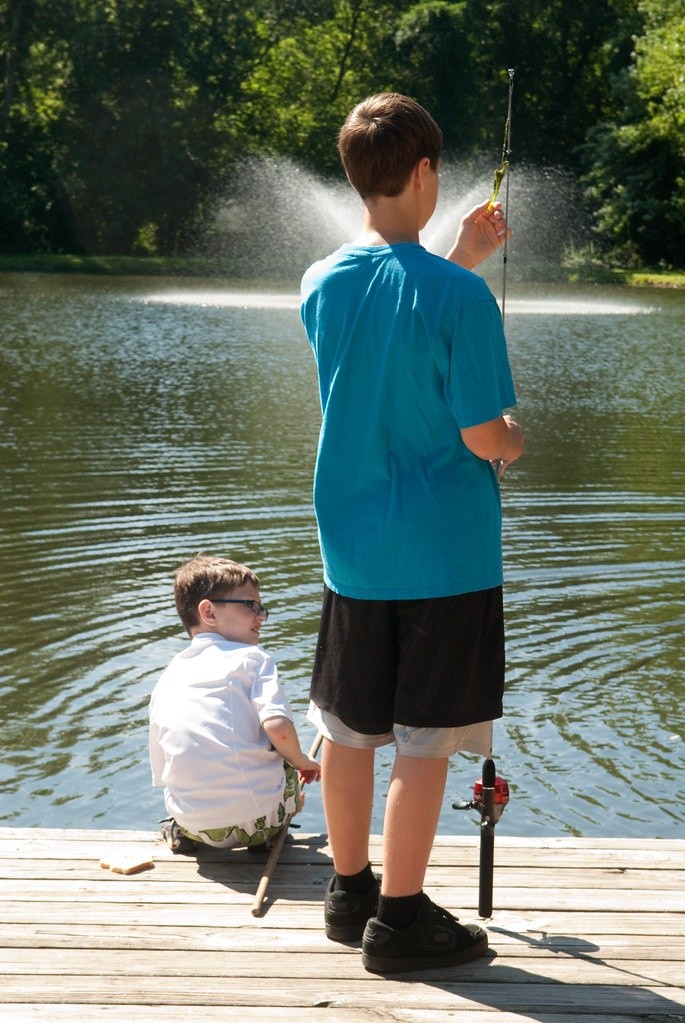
[146,555,321,854]
[296,91,526,973]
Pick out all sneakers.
[362,894,489,972]
[248,833,284,854]
[324,871,382,940]
[158,815,193,852]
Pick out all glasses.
[209,598,269,622]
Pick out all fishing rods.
[446,61,519,923]
[249,729,323,918]
